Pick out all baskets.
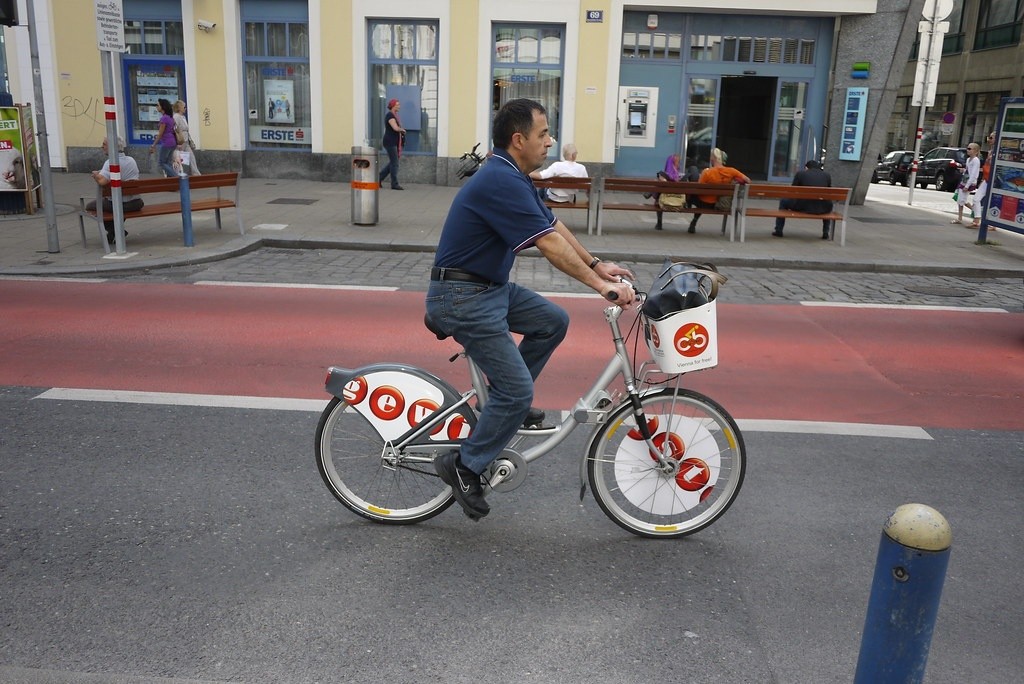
[636,297,718,375]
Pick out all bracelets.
[590,256,600,270]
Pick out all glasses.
[988,136,994,140]
[967,147,973,151]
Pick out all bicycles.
[456,143,485,180]
[313,274,748,541]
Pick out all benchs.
[530,174,596,237]
[597,176,740,243]
[735,177,853,246]
[77,169,244,255]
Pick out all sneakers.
[433,450,496,517]
[475,402,551,425]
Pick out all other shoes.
[950,218,964,224]
[379,181,382,188]
[772,230,784,237]
[655,224,663,230]
[642,192,652,199]
[390,184,406,191]
[687,225,697,233]
[107,231,128,243]
[966,221,980,229]
[987,226,997,231]
[823,233,830,239]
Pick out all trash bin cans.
[351,144,378,224]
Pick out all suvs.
[870,150,928,190]
[905,147,989,192]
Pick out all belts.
[428,266,498,290]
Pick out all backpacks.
[714,179,746,215]
[656,169,691,212]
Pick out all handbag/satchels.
[172,124,185,145]
[640,257,728,321]
[959,174,976,192]
[178,151,193,166]
[188,134,197,150]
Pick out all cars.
[686,126,713,163]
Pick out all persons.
[378,99,406,190]
[425,98,636,519]
[528,143,589,212]
[268,97,291,120]
[86,135,144,242]
[950,130,997,232]
[641,148,751,233]
[148,98,201,178]
[771,160,834,240]
